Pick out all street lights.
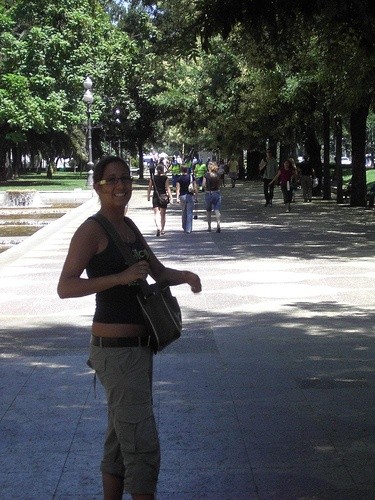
[82,76,95,191]
[116,109,121,158]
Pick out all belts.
[90,334,148,348]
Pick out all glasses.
[96,175,133,186]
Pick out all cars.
[341,157,352,164]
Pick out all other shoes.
[156,229,160,236]
[161,231,164,234]
[216,228,221,233]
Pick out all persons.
[57,156,203,500]
[176,166,198,234]
[147,157,225,192]
[227,156,240,187]
[147,164,173,237]
[258,149,316,212]
[202,162,222,233]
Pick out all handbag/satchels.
[209,192,220,204]
[258,158,267,175]
[313,178,319,188]
[136,280,182,353]
[188,173,195,193]
[158,194,170,204]
